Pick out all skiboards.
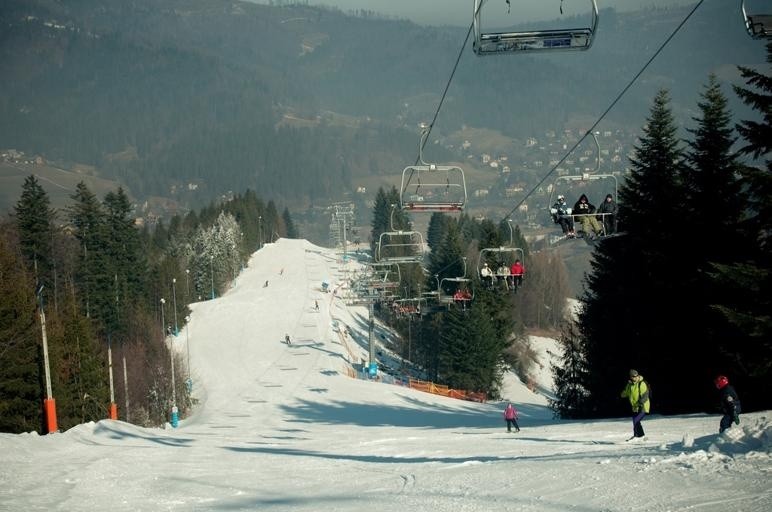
[500,431,522,433]
[487,289,522,295]
[592,437,649,444]
[552,233,623,249]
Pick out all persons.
[284,334,292,345]
[399,305,408,317]
[496,260,515,291]
[454,288,465,312]
[279,268,284,274]
[550,193,577,239]
[265,280,268,287]
[480,262,494,293]
[621,367,652,439]
[343,328,349,339]
[315,301,319,311]
[511,259,526,294]
[572,192,604,241]
[594,194,620,235]
[503,402,521,433]
[463,289,473,308]
[409,305,421,319]
[403,305,411,315]
[714,373,743,434]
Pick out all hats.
[629,368,638,378]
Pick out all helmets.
[716,375,728,388]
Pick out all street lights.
[211,255,214,299]
[258,215,261,249]
[241,232,243,271]
[232,245,236,285]
[160,268,192,429]
